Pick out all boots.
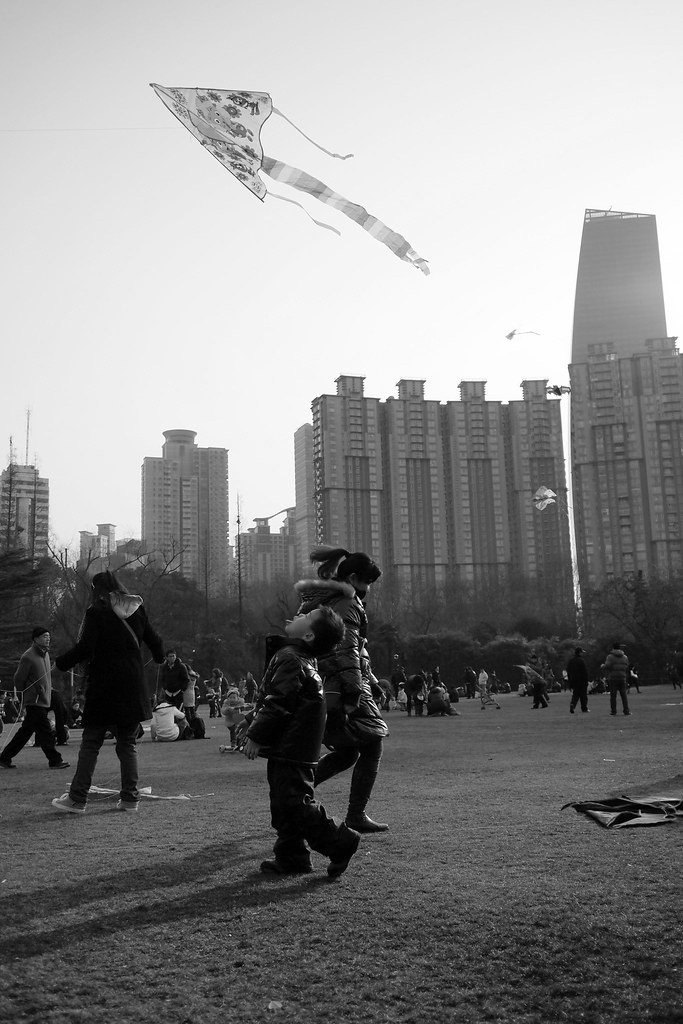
[313,754,347,788]
[346,769,388,832]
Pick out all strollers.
[472,681,502,710]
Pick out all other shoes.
[0,763,16,769]
[50,760,71,768]
[328,830,360,879]
[261,859,314,875]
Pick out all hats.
[32,627,50,643]
[227,689,240,696]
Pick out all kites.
[505,329,540,340]
[530,486,557,510]
[544,385,571,396]
[560,794,683,828]
[65,783,215,800]
[148,82,430,274]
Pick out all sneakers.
[117,798,138,811]
[51,793,86,813]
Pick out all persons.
[378,664,459,717]
[51,573,166,812]
[668,665,682,689]
[0,627,69,768]
[295,543,389,832]
[567,647,591,712]
[243,604,362,881]
[513,655,572,708]
[462,666,511,698]
[47,688,82,745]
[0,691,25,723]
[204,668,258,748]
[151,649,200,741]
[587,665,641,694]
[601,643,633,715]
